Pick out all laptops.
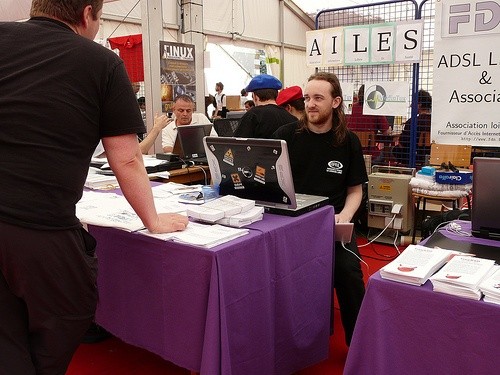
[172,122,212,159]
[469,156,500,240]
[202,136,330,219]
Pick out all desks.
[412,193,472,244]
[342,245,500,375]
[75,165,334,375]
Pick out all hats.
[246,74,282,92]
[276,86,303,106]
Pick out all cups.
[202,185,219,203]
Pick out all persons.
[0,0,187,375]
[94,73,433,352]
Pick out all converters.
[156,152,180,162]
